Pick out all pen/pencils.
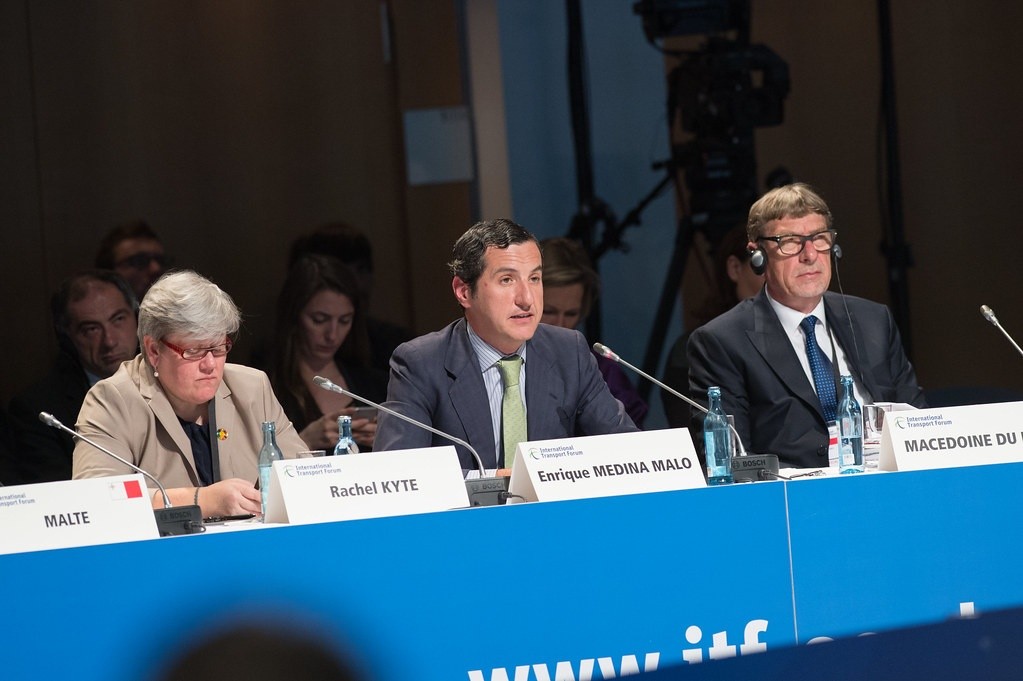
[203,514,256,521]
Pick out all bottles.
[704,387,734,486]
[334,416,359,455]
[258,422,284,523]
[836,377,864,475]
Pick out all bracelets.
[193,484,202,506]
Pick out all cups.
[719,415,736,458]
[863,406,892,467]
[296,451,325,459]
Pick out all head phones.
[749,244,843,275]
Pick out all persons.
[249,221,416,369]
[70,269,317,520]
[371,218,644,470]
[537,237,650,437]
[685,181,932,470]
[262,254,396,451]
[0,263,144,488]
[93,218,179,352]
[659,214,767,470]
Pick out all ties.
[800,315,847,427]
[495,356,528,468]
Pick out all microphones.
[312,376,506,508]
[980,304,1023,356]
[38,411,203,538]
[593,342,779,483]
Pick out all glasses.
[159,333,234,361]
[755,230,837,256]
[111,251,172,270]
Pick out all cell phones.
[353,405,376,420]
